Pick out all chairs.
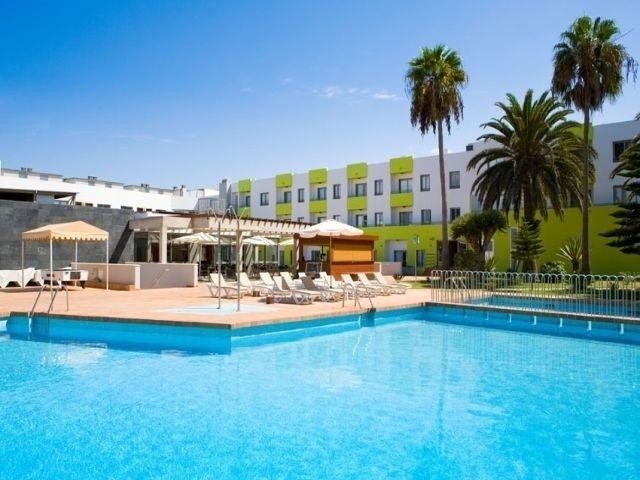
[207,272,412,305]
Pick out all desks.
[41,269,89,289]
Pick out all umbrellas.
[233,236,278,269]
[170,232,229,275]
[300,218,364,271]
[279,238,296,249]
[203,236,237,255]
[262,237,277,247]
